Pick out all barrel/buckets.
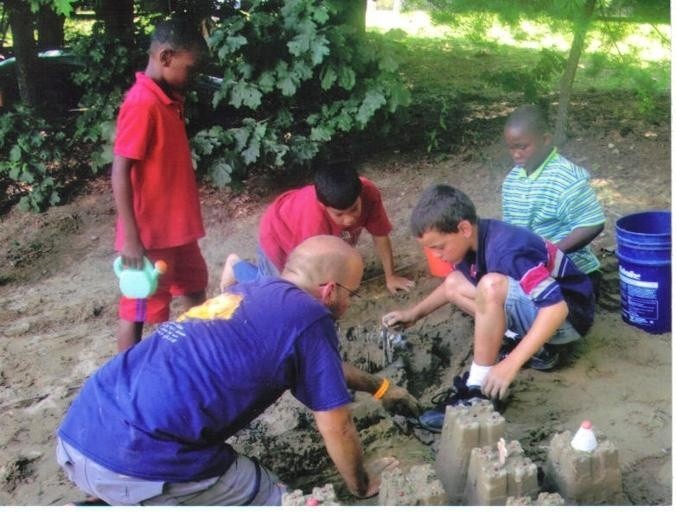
[613,210,671,335]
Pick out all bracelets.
[372,379,389,402]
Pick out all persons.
[54,234,425,505]
[380,182,597,438]
[218,159,418,296]
[499,104,610,315]
[109,17,210,351]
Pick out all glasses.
[319,282,361,300]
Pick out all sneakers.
[419,371,507,433]
[499,343,560,370]
